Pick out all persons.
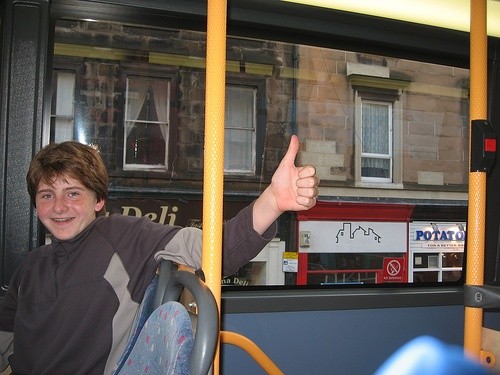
[0,135,321,375]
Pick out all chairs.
[112,270,218,375]
